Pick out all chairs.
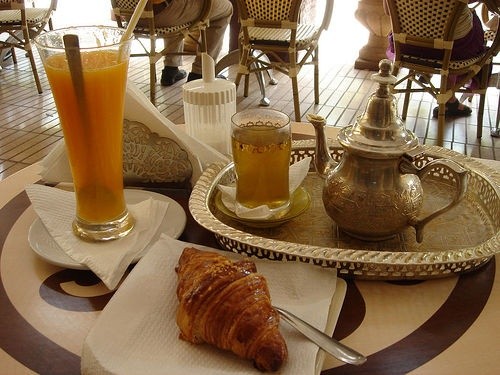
[234,0,334,122]
[385,0,500,149]
[0,0,58,95]
[111,0,215,107]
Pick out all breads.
[174,247,289,373]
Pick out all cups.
[34,25,135,241]
[230,108,292,218]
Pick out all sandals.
[419,75,433,84]
[433,99,472,118]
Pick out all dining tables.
[0,117,500,375]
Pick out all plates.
[215,184,310,229]
[88,278,347,374]
[27,189,189,269]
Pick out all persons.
[116,0,233,86]
[382,0,499,118]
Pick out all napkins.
[80,233,338,375]
[217,156,313,223]
[22,181,171,292]
[38,78,230,186]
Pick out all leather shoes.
[187,72,227,82]
[160,69,186,85]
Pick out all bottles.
[182,53,237,155]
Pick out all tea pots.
[306,58,470,242]
